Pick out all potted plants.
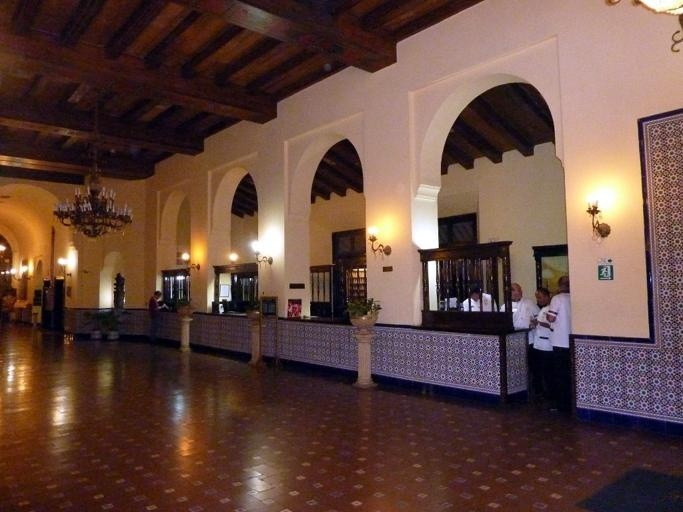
[344,297,383,332]
[83,306,120,340]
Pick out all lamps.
[51,85,134,239]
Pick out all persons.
[549,275,573,418]
[526,288,556,412]
[462,283,498,313]
[500,282,538,332]
[148,289,167,343]
